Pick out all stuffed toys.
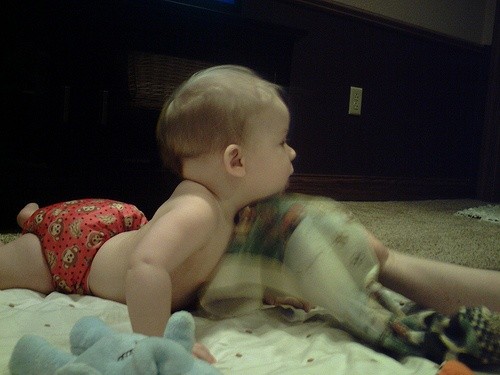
[7,310,223,375]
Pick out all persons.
[361,225,500,317]
[0,64,297,366]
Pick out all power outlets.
[348,86,362,115]
